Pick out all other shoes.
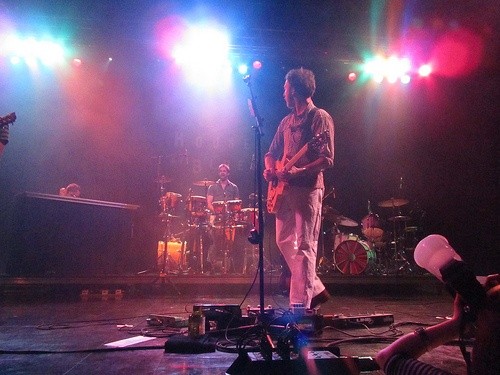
[311,289,329,308]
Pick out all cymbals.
[377,198,410,207]
[323,204,359,227]
[192,180,216,187]
[153,177,175,184]
[388,215,412,222]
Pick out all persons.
[375,274,500,375]
[0,125,9,155]
[64,184,80,197]
[207,164,239,243]
[264,70,334,308]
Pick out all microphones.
[333,188,335,199]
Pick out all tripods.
[316,192,332,271]
[382,207,413,275]
[206,74,294,351]
[142,209,184,277]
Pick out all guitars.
[266,129,330,214]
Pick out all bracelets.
[417,328,433,351]
[303,168,306,174]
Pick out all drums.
[333,238,374,278]
[211,199,243,229]
[362,214,384,238]
[373,241,388,270]
[163,191,183,218]
[185,195,208,228]
[403,226,424,251]
[241,207,260,236]
